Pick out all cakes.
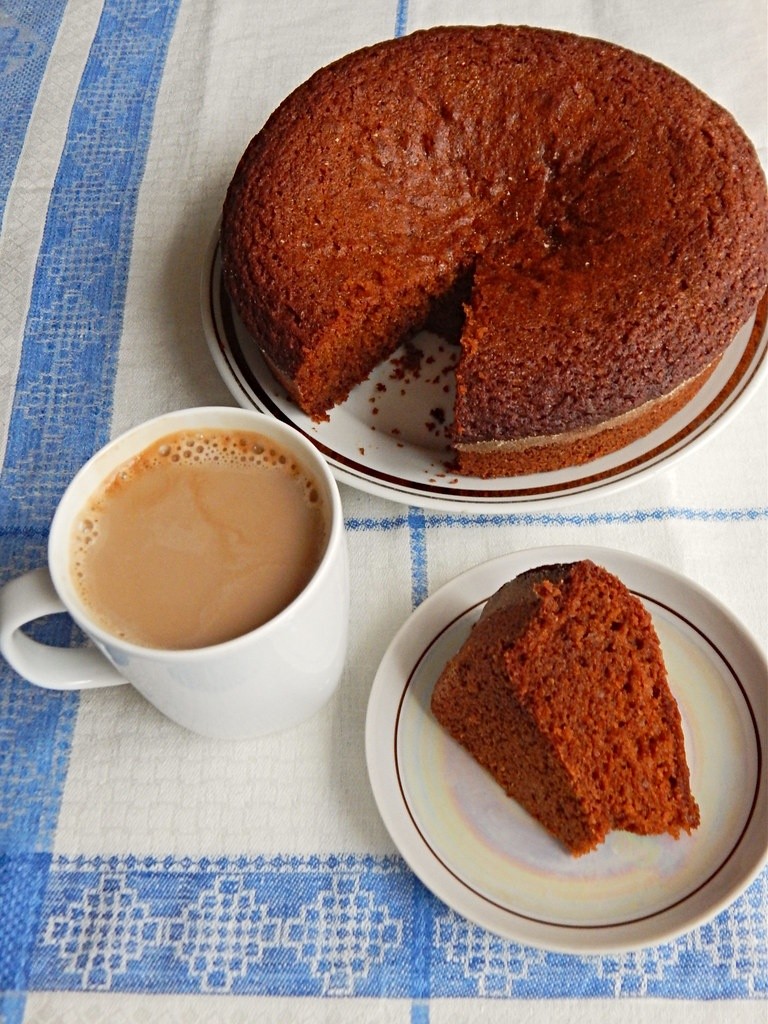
[431,559,702,857]
[223,25,768,479]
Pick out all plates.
[200,211,768,511]
[365,545,768,956]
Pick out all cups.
[0,405,349,740]
